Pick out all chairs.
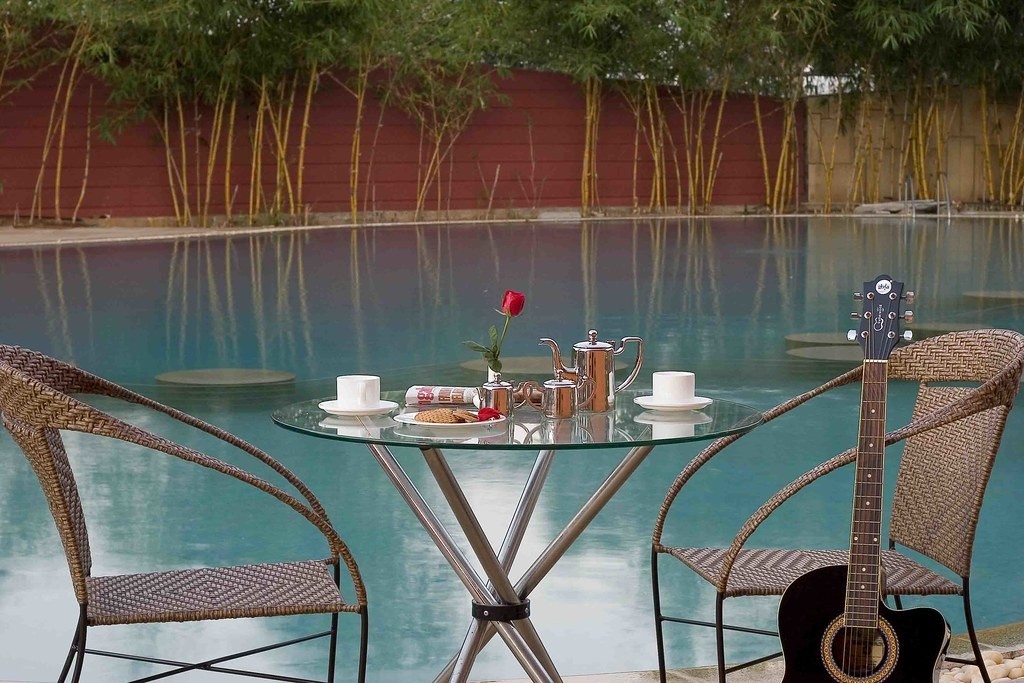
[653,329,1024,683]
[0,338,370,683]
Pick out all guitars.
[776,274,954,682]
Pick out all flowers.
[462,290,525,372]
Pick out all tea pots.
[524,369,597,418]
[538,329,643,413]
[476,373,533,417]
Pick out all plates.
[633,395,713,412]
[633,410,713,425]
[318,416,399,429]
[318,400,399,416]
[393,425,506,440]
[393,411,506,427]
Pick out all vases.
[487,367,502,384]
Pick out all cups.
[336,429,380,439]
[652,371,695,403]
[652,424,694,440]
[336,375,380,408]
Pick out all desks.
[272,395,763,683]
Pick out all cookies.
[414,408,479,423]
